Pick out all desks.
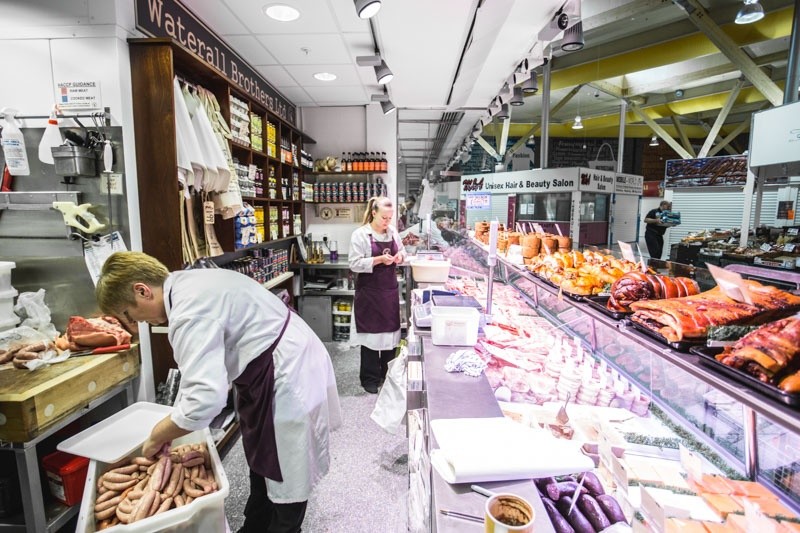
[0,339,143,533]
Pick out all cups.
[484,493,536,533]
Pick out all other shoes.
[364,384,378,393]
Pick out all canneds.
[332,301,351,337]
[221,248,289,284]
[313,182,387,202]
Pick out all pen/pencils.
[471,484,494,497]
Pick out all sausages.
[0,343,45,369]
[535,473,628,533]
[94,442,219,531]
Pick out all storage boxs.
[411,258,451,283]
[430,305,481,348]
[42,421,231,533]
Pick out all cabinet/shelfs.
[127,33,409,463]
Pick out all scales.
[412,220,498,328]
[416,213,444,261]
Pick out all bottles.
[280,135,292,163]
[341,151,387,171]
[250,111,276,158]
[281,178,290,199]
[300,149,313,170]
[777,232,794,244]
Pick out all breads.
[473,221,570,267]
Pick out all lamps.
[497,103,510,120]
[373,57,395,84]
[560,20,585,52]
[571,92,584,130]
[513,61,532,85]
[352,0,382,20]
[443,115,493,173]
[499,84,515,105]
[526,118,536,145]
[521,70,539,94]
[524,43,545,71]
[488,96,503,116]
[648,129,659,147]
[734,0,766,24]
[379,98,397,115]
[510,86,525,106]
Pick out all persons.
[348,196,451,393]
[644,200,677,258]
[96,251,344,533]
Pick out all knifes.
[70,344,130,356]
